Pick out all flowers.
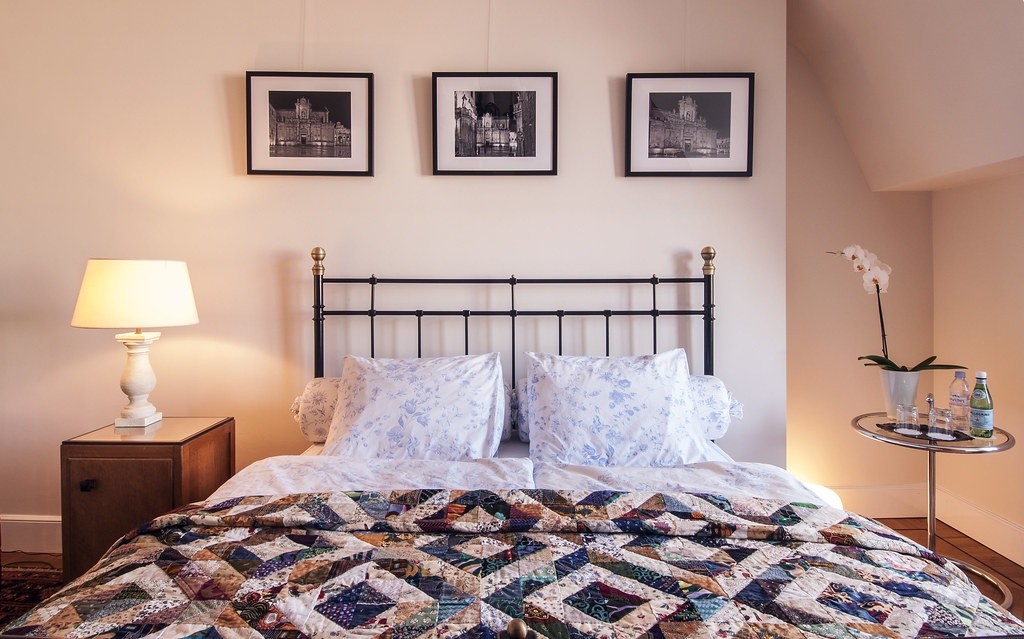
[824,244,970,371]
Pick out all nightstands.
[60,413,236,588]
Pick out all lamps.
[71,257,200,428]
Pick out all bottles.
[970,371,993,438]
[950,372,970,431]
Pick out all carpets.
[0,567,63,632]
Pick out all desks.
[850,412,1016,609]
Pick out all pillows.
[523,348,711,467]
[320,351,505,458]
[291,378,515,444]
[509,374,744,442]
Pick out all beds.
[0,243,1022,639]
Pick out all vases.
[881,370,921,419]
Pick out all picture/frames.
[432,72,560,176]
[624,72,755,177]
[245,72,376,176]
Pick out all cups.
[895,404,920,433]
[928,408,953,438]
